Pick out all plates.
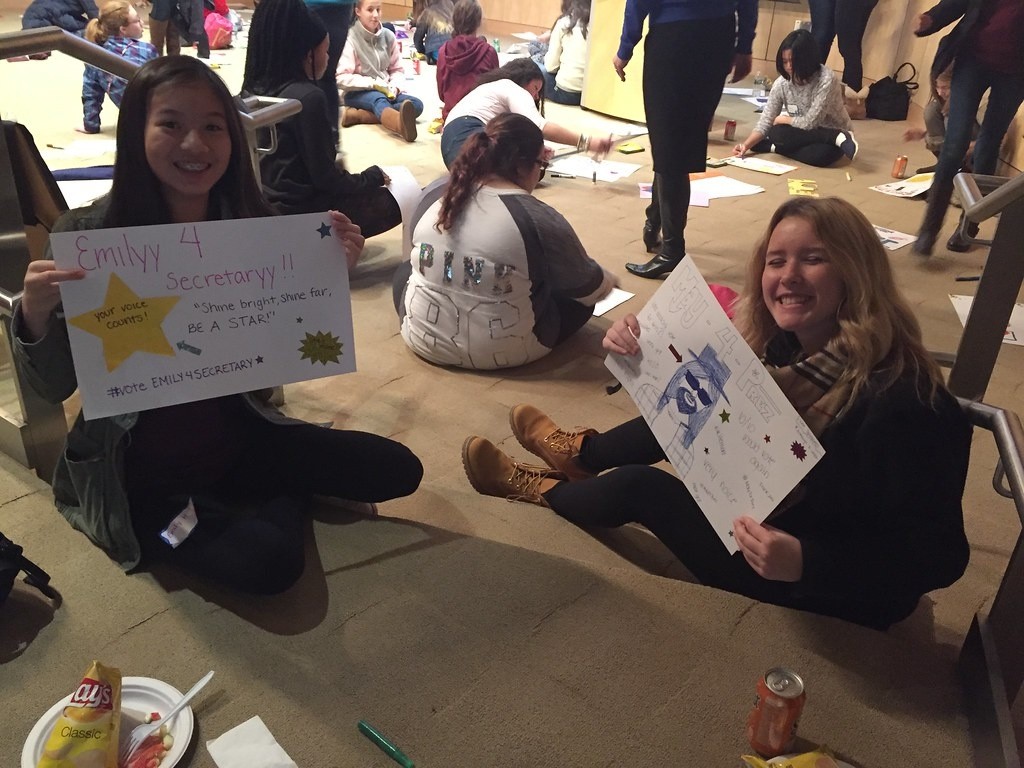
[21,677,194,768]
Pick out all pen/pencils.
[592,171,596,185]
[358,720,415,768]
[845,172,852,181]
[47,144,64,149]
[739,144,744,160]
[551,174,577,179]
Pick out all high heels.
[643,226,661,252]
[625,253,678,279]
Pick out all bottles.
[752,70,763,97]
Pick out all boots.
[914,208,946,254]
[149,13,168,57]
[916,135,944,173]
[843,84,870,119]
[341,106,381,128]
[381,99,417,142]
[165,18,181,56]
[947,211,980,251]
[189,28,210,60]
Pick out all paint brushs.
[954,276,980,281]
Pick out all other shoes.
[840,130,859,161]
[751,140,772,154]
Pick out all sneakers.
[461,435,569,510]
[509,403,602,483]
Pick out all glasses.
[124,15,140,24]
[517,154,549,171]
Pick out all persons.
[732,30,859,167]
[441,59,613,175]
[334,0,424,141]
[808,0,879,91]
[903,62,990,174]
[11,0,425,595]
[533,0,591,107]
[613,0,759,278]
[460,196,971,635]
[409,0,498,121]
[910,0,1024,260]
[390,113,621,368]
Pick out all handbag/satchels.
[194,13,234,50]
[867,62,919,121]
[226,9,244,32]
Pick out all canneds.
[412,59,420,75]
[397,41,402,53]
[748,667,806,758]
[891,155,908,178]
[492,38,500,53]
[724,120,736,140]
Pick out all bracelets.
[577,134,591,152]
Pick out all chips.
[46,743,106,768]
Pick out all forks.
[118,670,214,766]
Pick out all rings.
[615,69,617,70]
